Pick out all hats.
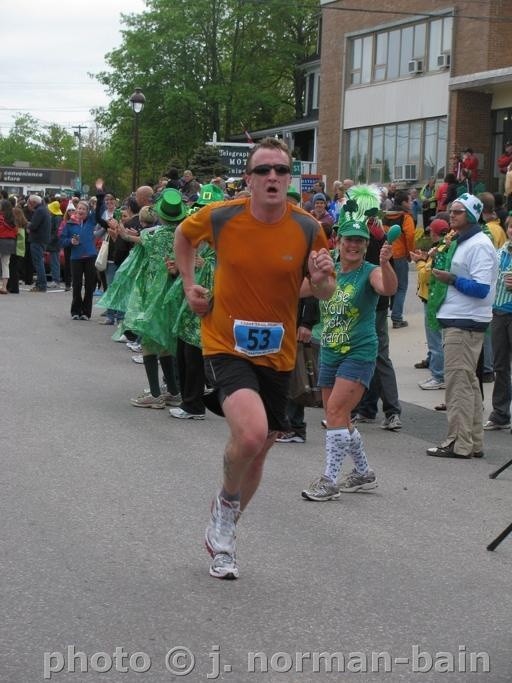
[451,191,484,223]
[425,218,450,234]
[156,187,188,220]
[191,184,225,207]
[336,220,370,240]
[430,211,450,223]
[459,146,472,152]
[166,168,180,180]
[48,200,64,215]
[287,185,301,202]
[312,193,326,204]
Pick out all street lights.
[128,86,146,194]
[71,126,88,188]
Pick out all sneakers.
[72,314,80,319]
[414,359,511,458]
[322,412,402,429]
[392,319,408,327]
[0,274,72,293]
[204,493,242,554]
[99,309,161,364]
[80,314,89,320]
[129,383,206,420]
[275,426,305,443]
[301,473,341,500]
[337,468,378,492]
[209,555,239,578]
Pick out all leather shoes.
[96,289,105,295]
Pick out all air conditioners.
[437,55,450,67]
[392,161,420,185]
[408,59,423,75]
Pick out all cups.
[502,269,512,291]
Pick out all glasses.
[247,163,290,174]
[449,208,466,214]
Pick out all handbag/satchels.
[16,227,27,258]
[288,341,323,407]
[95,232,112,271]
